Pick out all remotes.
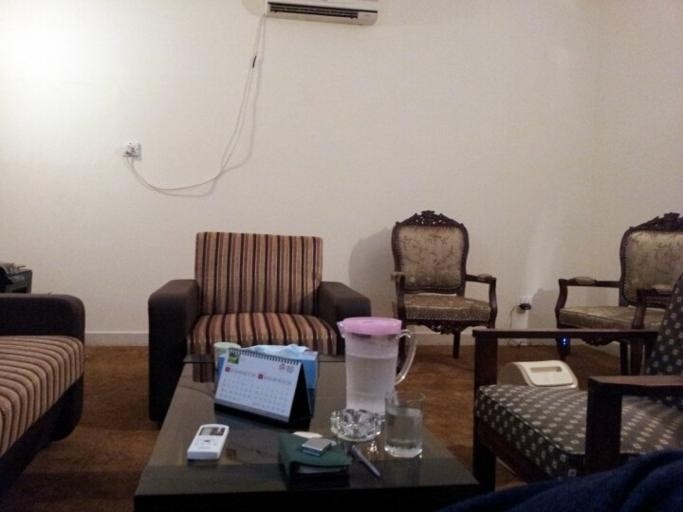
[187,424,228,462]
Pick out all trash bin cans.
[501,358,581,392]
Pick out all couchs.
[0,292,86,496]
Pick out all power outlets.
[124,142,141,157]
[518,296,532,312]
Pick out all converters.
[520,304,532,309]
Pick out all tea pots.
[336,316,417,424]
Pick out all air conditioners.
[264,0,378,26]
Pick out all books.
[277,432,352,476]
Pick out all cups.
[385,389,426,459]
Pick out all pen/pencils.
[351,444,382,477]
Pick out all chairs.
[147,232,372,423]
[391,211,498,359]
[559,211,683,375]
[472,270,682,491]
